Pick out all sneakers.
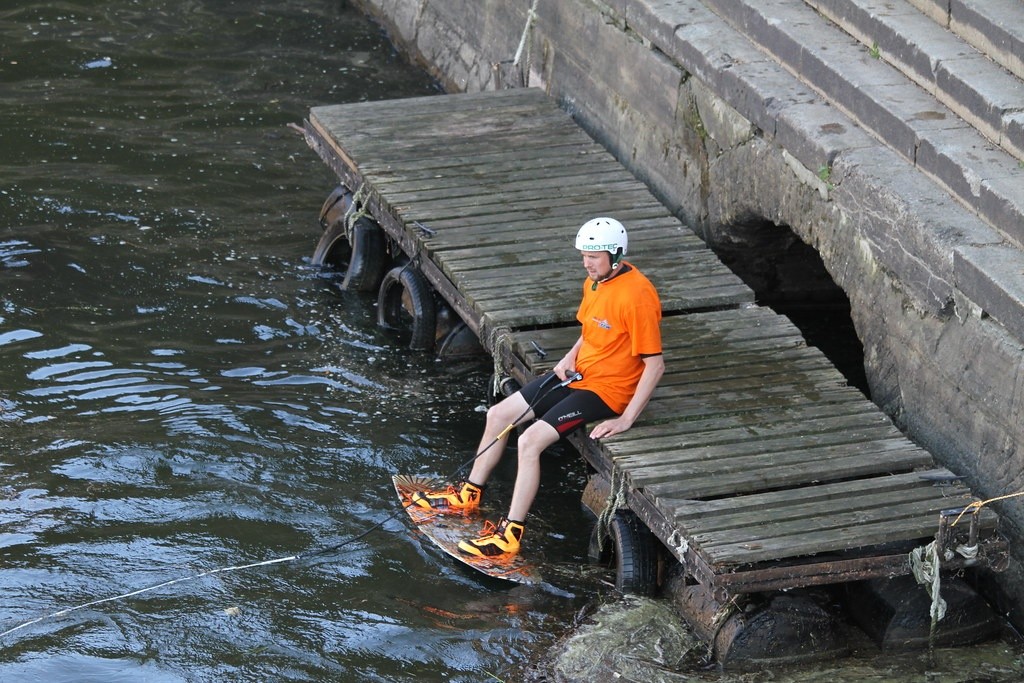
[457,518,525,561]
[411,479,482,516]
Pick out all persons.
[412,217,665,560]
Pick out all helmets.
[575,217,628,256]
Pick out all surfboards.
[392,474,543,585]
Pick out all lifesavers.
[588,506,658,600]
[375,265,436,352]
[310,214,385,293]
